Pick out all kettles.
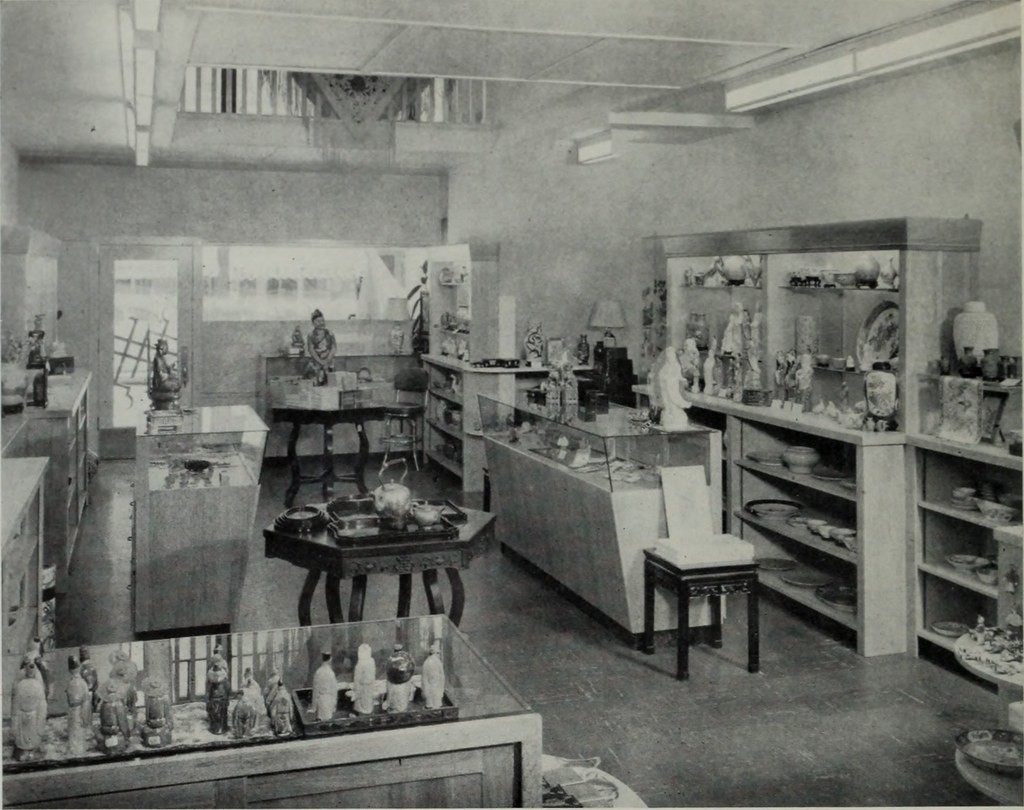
[369,458,410,528]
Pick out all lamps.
[382,298,413,353]
[588,297,626,348]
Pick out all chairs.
[378,366,429,474]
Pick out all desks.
[953,621,1024,730]
[269,403,388,509]
[953,747,1024,806]
[263,497,497,628]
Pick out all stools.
[639,548,762,679]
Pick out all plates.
[930,620,967,637]
[788,517,809,528]
[857,301,900,366]
[747,449,786,465]
[781,570,832,586]
[815,582,856,613]
[746,500,803,521]
[946,554,989,572]
[755,558,797,570]
[955,728,1024,778]
[813,470,853,480]
[951,498,978,510]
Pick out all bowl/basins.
[808,519,828,533]
[977,568,998,584]
[817,525,836,539]
[828,528,856,544]
[833,274,856,287]
[952,487,975,500]
[819,270,839,284]
[843,537,855,550]
[998,494,1022,509]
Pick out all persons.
[153,339,181,392]
[12,636,446,762]
[660,303,855,419]
[303,309,337,386]
[292,324,304,342]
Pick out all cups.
[816,354,831,365]
[831,358,846,368]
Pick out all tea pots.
[411,501,445,527]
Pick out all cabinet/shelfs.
[29,368,90,594]
[2,456,44,652]
[631,215,981,657]
[903,431,1024,655]
[419,239,501,494]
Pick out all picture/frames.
[980,389,1009,444]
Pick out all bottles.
[959,347,977,378]
[981,349,1012,381]
[460,266,468,282]
[1007,609,1023,640]
[938,355,950,375]
